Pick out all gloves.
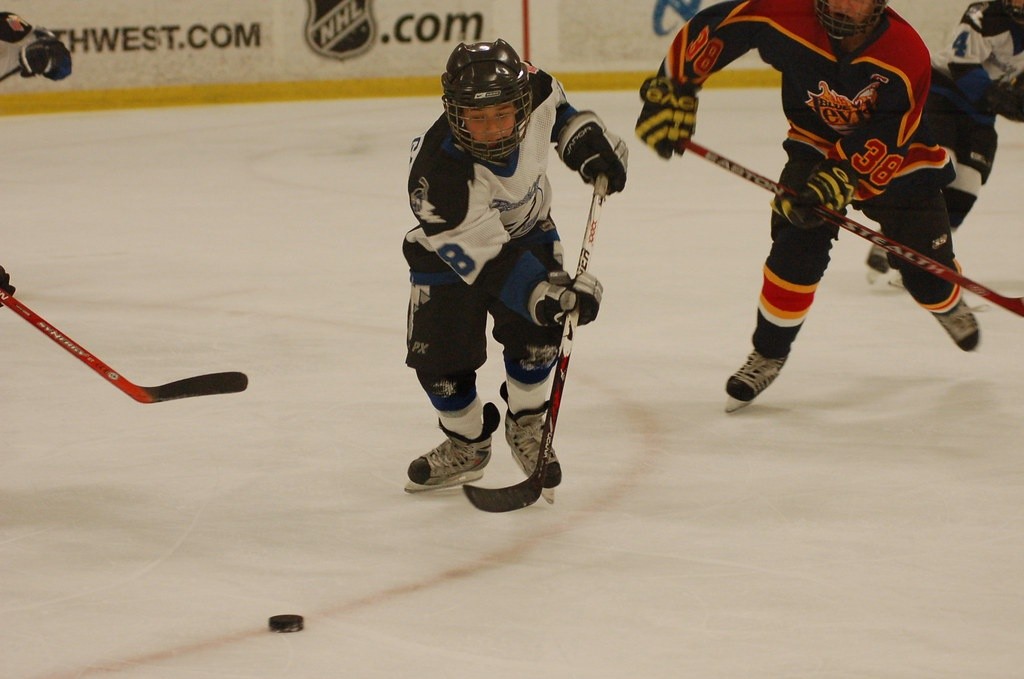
[17,37,73,79]
[978,71,1024,124]
[555,110,629,195]
[1,266,15,309]
[632,78,703,160]
[769,158,859,230]
[526,269,604,330]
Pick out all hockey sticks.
[0,288,249,404]
[685,140,1023,318]
[461,172,609,513]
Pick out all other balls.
[268,614,304,634]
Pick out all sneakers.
[932,299,993,351]
[724,335,791,413]
[865,244,891,284]
[499,380,563,505]
[402,402,501,493]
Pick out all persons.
[404,36,630,503]
[861,0,1024,291]
[0,9,73,308]
[634,0,983,413]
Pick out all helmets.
[817,0,889,24]
[440,37,533,110]
